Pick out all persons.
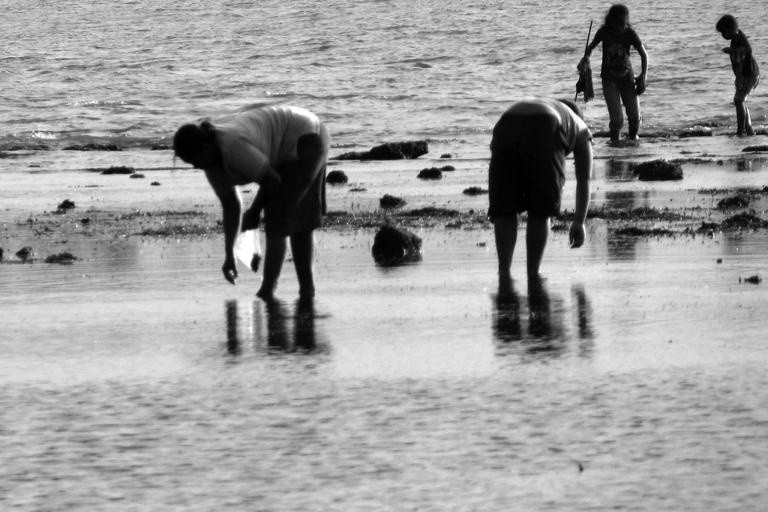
[490,281,594,362]
[724,150,757,256]
[608,145,643,265]
[486,98,592,281]
[223,301,315,357]
[576,4,649,142]
[714,14,760,134]
[170,102,328,301]
